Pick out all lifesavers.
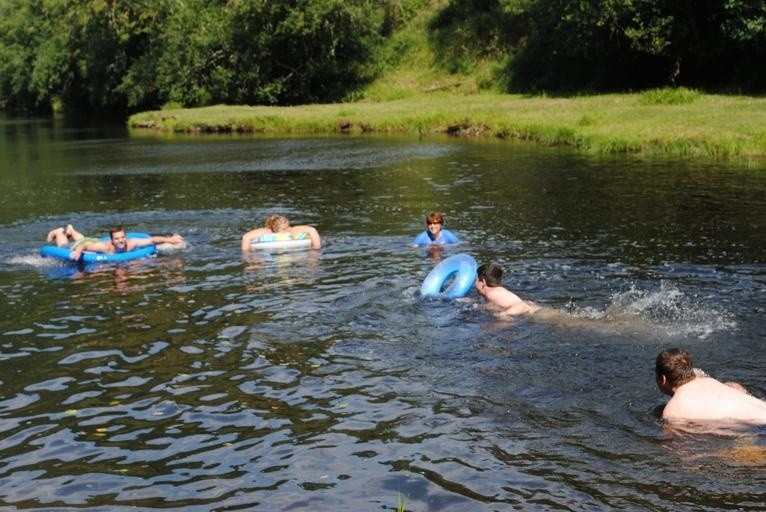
[251,231,312,252]
[40,231,157,267]
[419,254,479,303]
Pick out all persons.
[412,211,460,247]
[45,223,183,262]
[654,346,765,425]
[455,264,541,317]
[240,215,322,253]
